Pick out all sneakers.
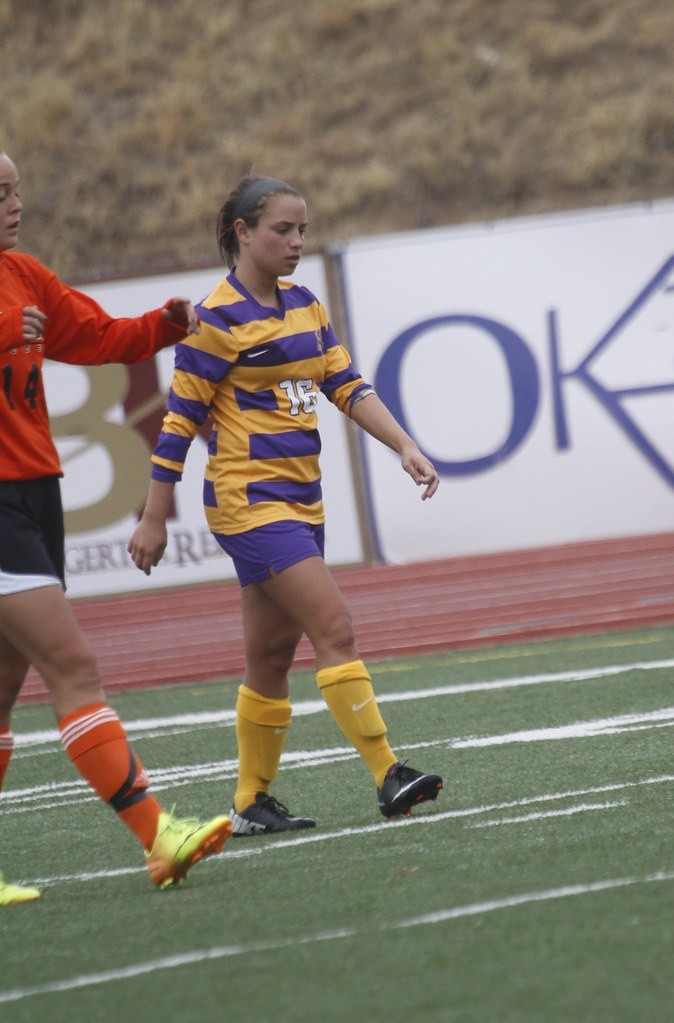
[144,802,233,891]
[229,791,316,837]
[0,881,41,906]
[376,758,443,818]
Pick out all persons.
[0,151,230,904]
[129,175,444,839]
